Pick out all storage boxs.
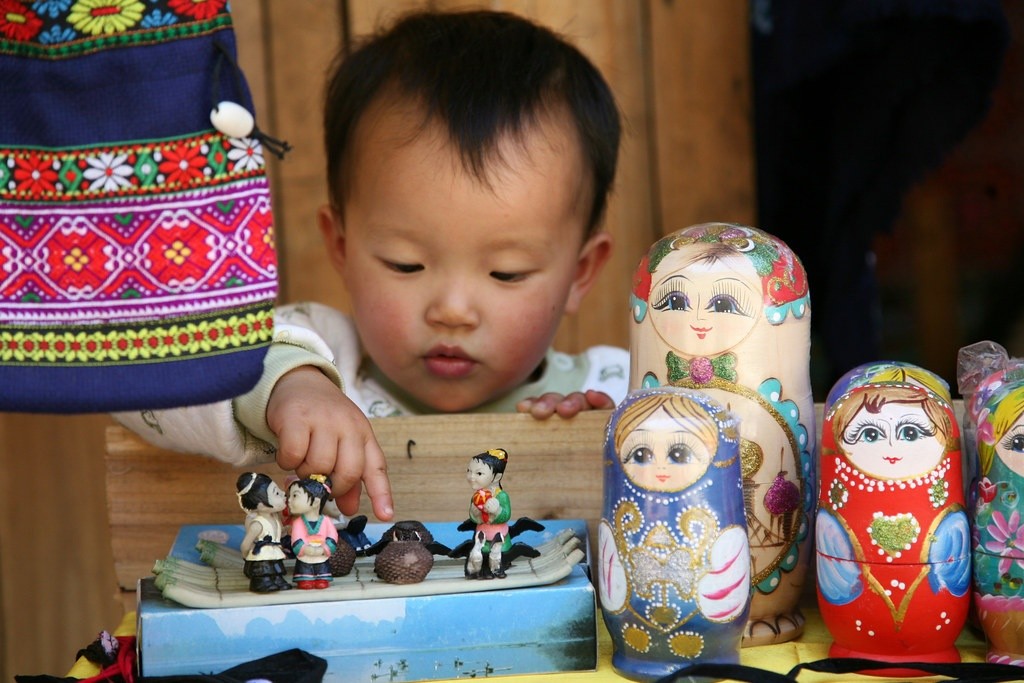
[167,520,595,583]
[137,562,598,683]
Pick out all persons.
[288,474,339,590]
[113,9,632,520]
[466,449,513,577]
[236,472,292,592]
